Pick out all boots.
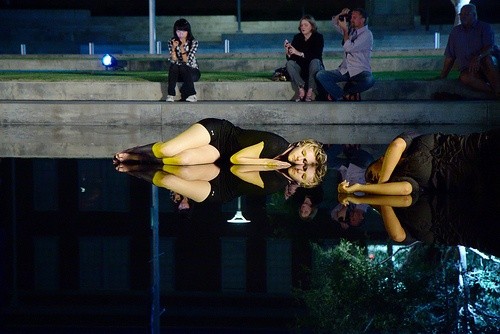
[114,141,163,160]
[154,164,165,170]
[154,160,164,166]
[127,169,160,185]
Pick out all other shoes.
[349,92,360,102]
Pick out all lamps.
[102,53,116,69]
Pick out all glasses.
[458,12,473,16]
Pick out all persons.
[333,206,500,257]
[115,165,326,221]
[337,129,500,195]
[317,8,375,101]
[437,4,500,95]
[167,18,201,102]
[284,15,325,103]
[114,118,327,173]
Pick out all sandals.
[296,93,306,102]
[304,97,313,102]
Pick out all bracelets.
[480,54,483,58]
[300,52,303,57]
[181,51,186,55]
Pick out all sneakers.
[166,94,175,102]
[185,95,197,103]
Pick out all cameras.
[339,13,351,22]
